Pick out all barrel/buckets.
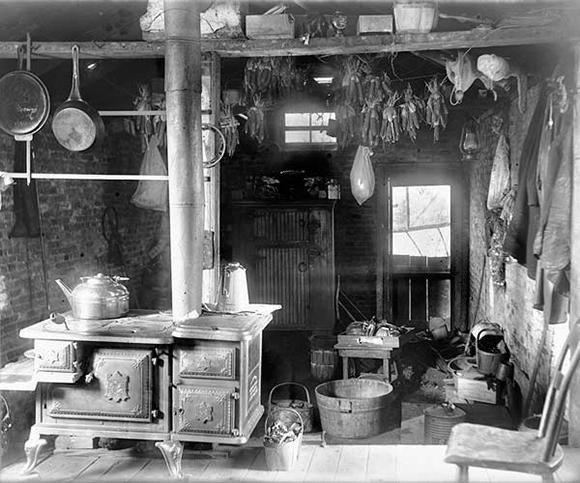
[309,334,338,383]
[519,415,568,444]
[475,328,509,376]
[422,404,467,445]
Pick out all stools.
[333,342,403,389]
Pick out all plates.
[423,406,467,440]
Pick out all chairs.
[444,319,579,482]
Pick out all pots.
[0,47,51,143]
[51,44,106,156]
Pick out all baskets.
[263,382,314,472]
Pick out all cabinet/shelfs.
[18,308,273,478]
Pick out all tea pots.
[217,261,250,315]
[55,273,131,320]
